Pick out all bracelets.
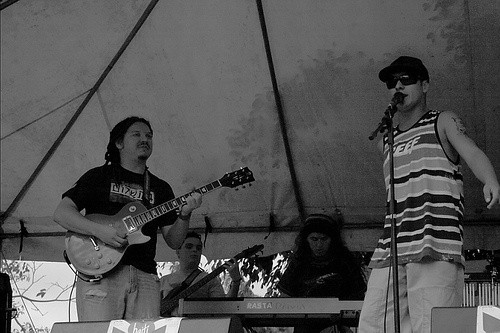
[233,278,242,282]
[180,209,192,220]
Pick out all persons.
[53,117,203,322]
[276,214,367,333]
[358,57,500,333]
[158,231,242,317]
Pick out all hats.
[378,56,429,83]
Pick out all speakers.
[431,306,500,333]
[48,316,244,333]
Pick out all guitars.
[160,243,265,316]
[64,166,256,277]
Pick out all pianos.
[178,297,362,320]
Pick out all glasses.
[178,243,202,251]
[385,74,424,89]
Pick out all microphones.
[388,92,404,110]
